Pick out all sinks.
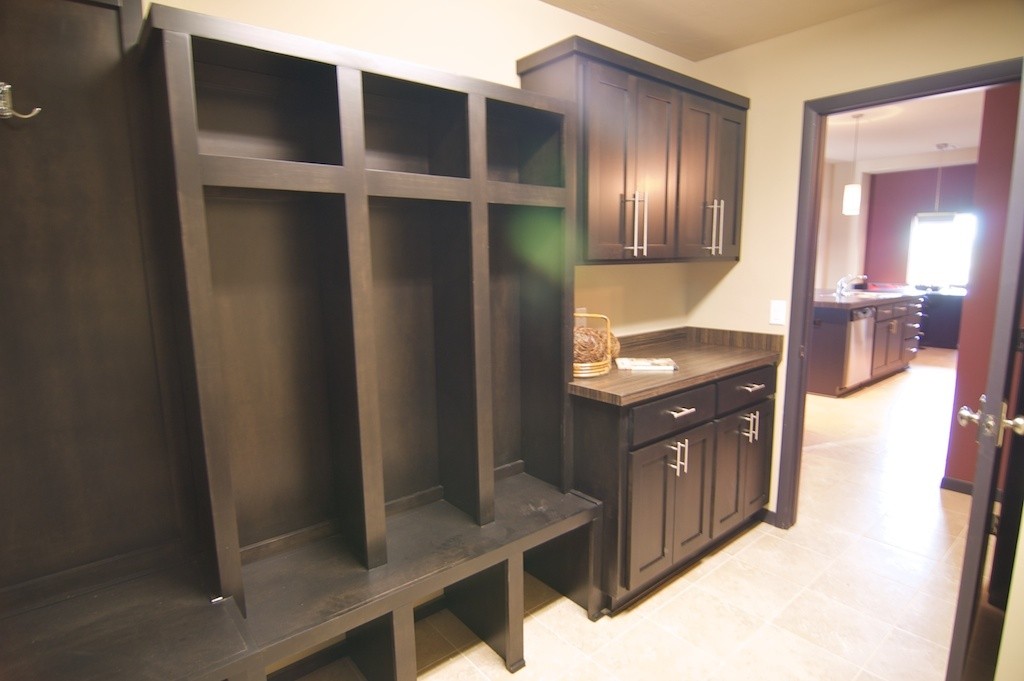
[850,292,903,299]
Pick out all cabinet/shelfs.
[517,35,750,267]
[867,297,923,375]
[138,2,604,681]
[523,362,777,623]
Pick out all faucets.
[836,274,868,297]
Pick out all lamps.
[917,143,955,224]
[842,113,865,215]
